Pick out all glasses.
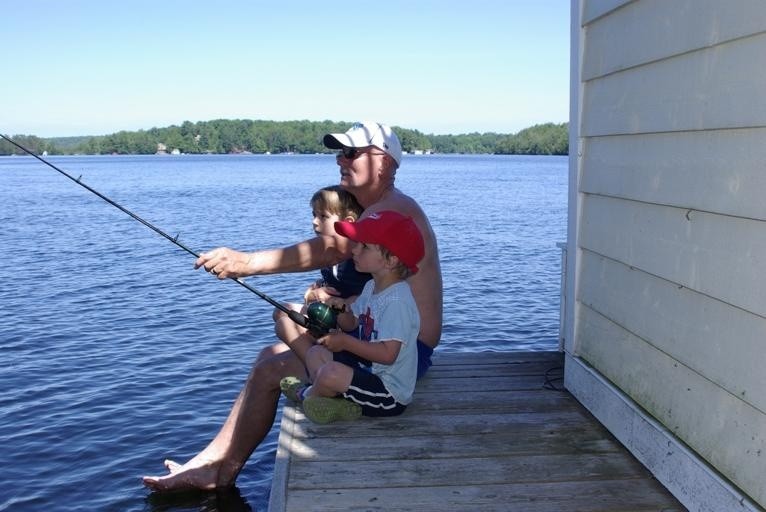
[336,149,386,159]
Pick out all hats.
[323,122,402,168]
[335,211,425,272]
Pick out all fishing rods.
[0,132,338,340]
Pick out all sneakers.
[280,376,362,424]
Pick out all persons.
[274,211,425,425]
[143,120,444,492]
[273,186,374,361]
